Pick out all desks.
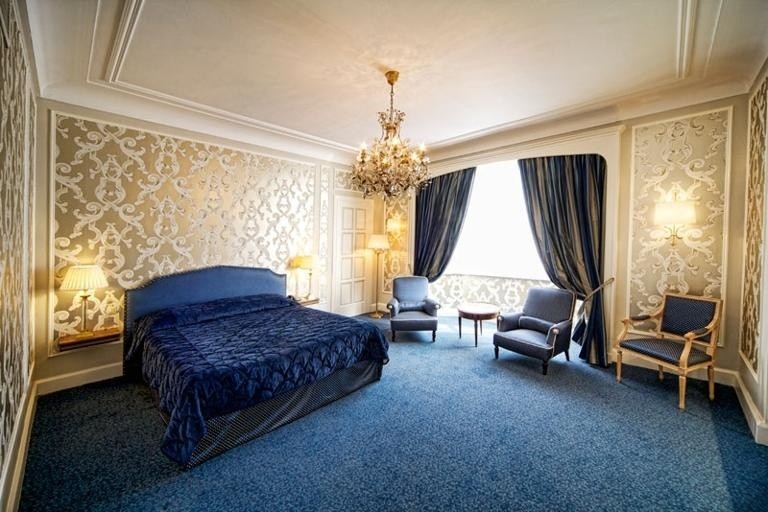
[455,301,500,346]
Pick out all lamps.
[287,254,319,299]
[59,262,111,339]
[368,233,391,320]
[348,70,434,202]
[649,189,700,245]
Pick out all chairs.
[491,284,578,376]
[388,274,441,345]
[614,285,722,410]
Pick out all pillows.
[518,314,556,335]
[398,300,426,312]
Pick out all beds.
[119,262,391,471]
